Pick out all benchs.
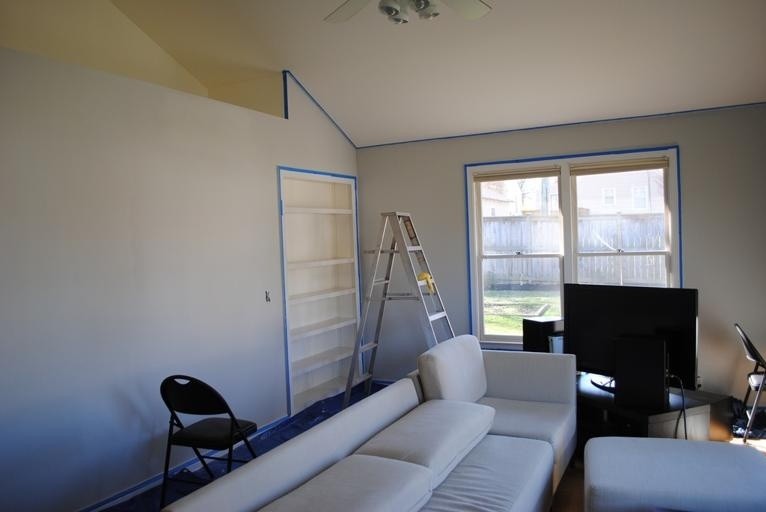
[584,437,766,510]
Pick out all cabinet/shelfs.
[278,166,365,417]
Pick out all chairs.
[734,322,766,441]
[160,374,257,507]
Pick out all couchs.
[159,350,576,510]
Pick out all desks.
[577,370,711,454]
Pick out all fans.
[323,0,491,23]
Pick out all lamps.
[378,0,439,23]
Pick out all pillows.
[253,453,435,510]
[353,399,498,488]
[417,333,489,403]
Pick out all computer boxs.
[523,315,564,352]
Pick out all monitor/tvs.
[562,282,699,400]
